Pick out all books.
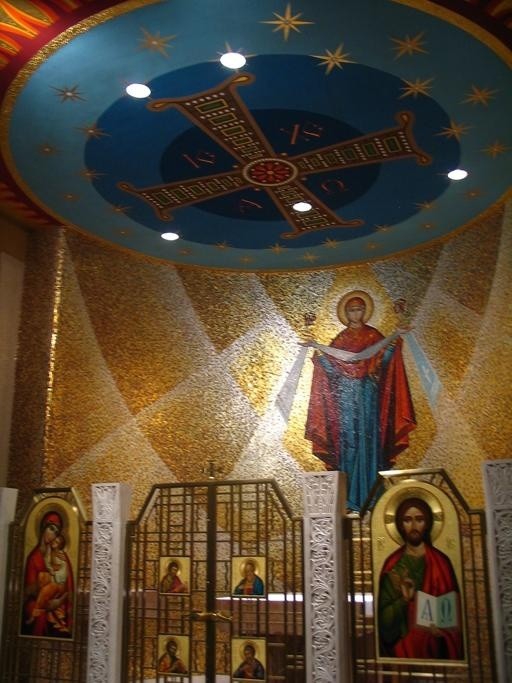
[415,586,459,631]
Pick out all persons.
[160,560,189,595]
[24,534,71,635]
[298,290,416,518]
[21,510,75,638]
[378,497,466,661]
[232,644,265,679]
[231,557,265,595]
[157,638,189,674]
